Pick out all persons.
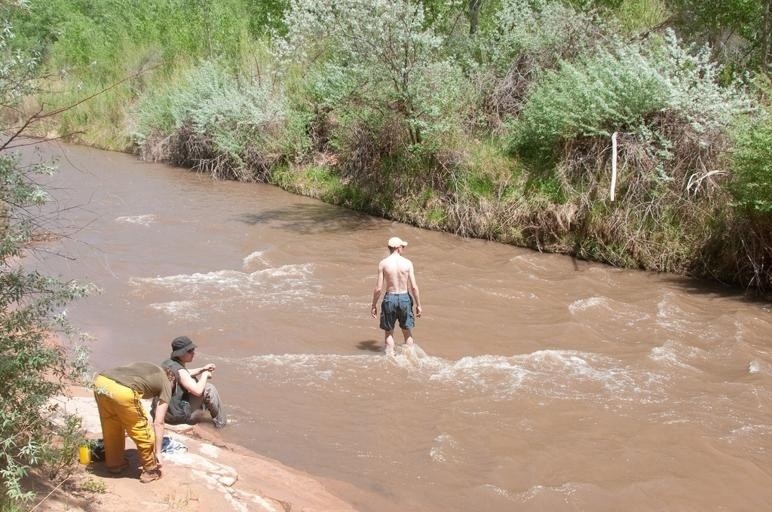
[371,236,422,355]
[150,337,226,429]
[93,360,177,483]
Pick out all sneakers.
[107,457,131,473]
[139,469,162,483]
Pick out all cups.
[79,444,92,464]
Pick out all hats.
[388,236,408,249]
[169,335,197,358]
